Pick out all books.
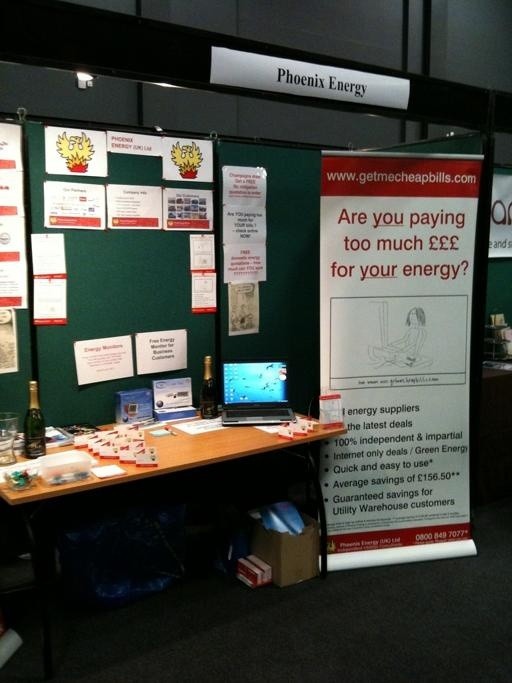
[9,421,99,458]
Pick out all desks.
[0,412,347,683]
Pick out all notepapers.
[149,429,169,437]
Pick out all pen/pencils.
[164,426,177,436]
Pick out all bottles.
[200,356,218,419]
[23,381,46,459]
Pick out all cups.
[0,412,19,467]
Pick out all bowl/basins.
[2,467,39,491]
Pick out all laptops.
[221,357,298,427]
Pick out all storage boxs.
[255,516,320,590]
